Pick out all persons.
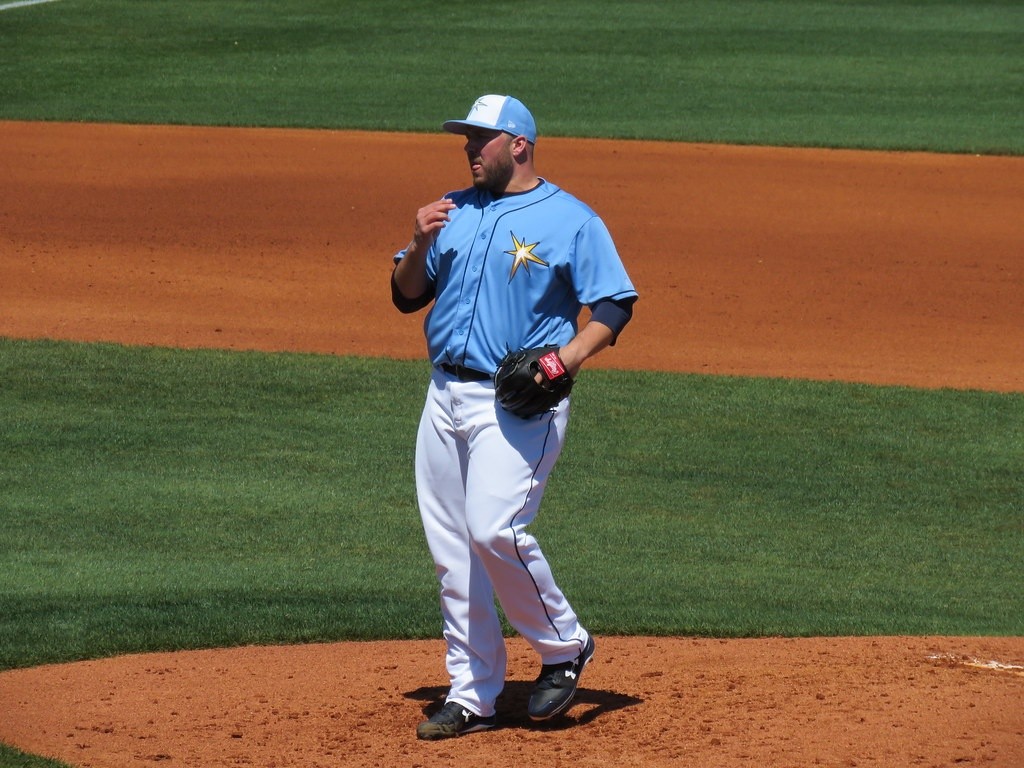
[382,97,643,739]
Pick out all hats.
[441,93,535,143]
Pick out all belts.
[434,363,496,380]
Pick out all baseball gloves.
[492,342,575,421]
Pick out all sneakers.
[528,636,596,721]
[417,701,498,738]
